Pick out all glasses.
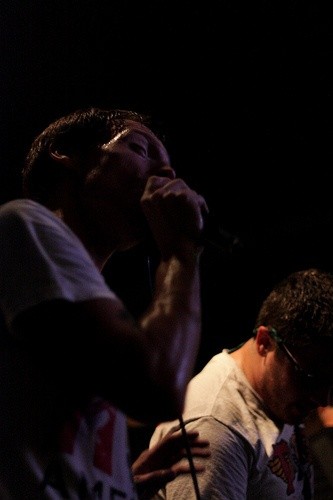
[254,326,333,391]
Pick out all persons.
[0,109,210,500]
[149,269,333,500]
